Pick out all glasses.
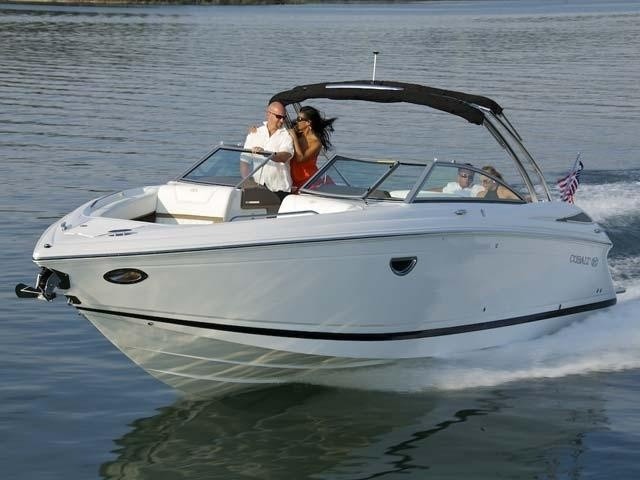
[459,174,473,179]
[297,116,308,122]
[268,111,287,120]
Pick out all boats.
[16,51,625,400]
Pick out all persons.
[239,101,295,202]
[475,166,520,200]
[426,163,488,197]
[247,106,338,190]
